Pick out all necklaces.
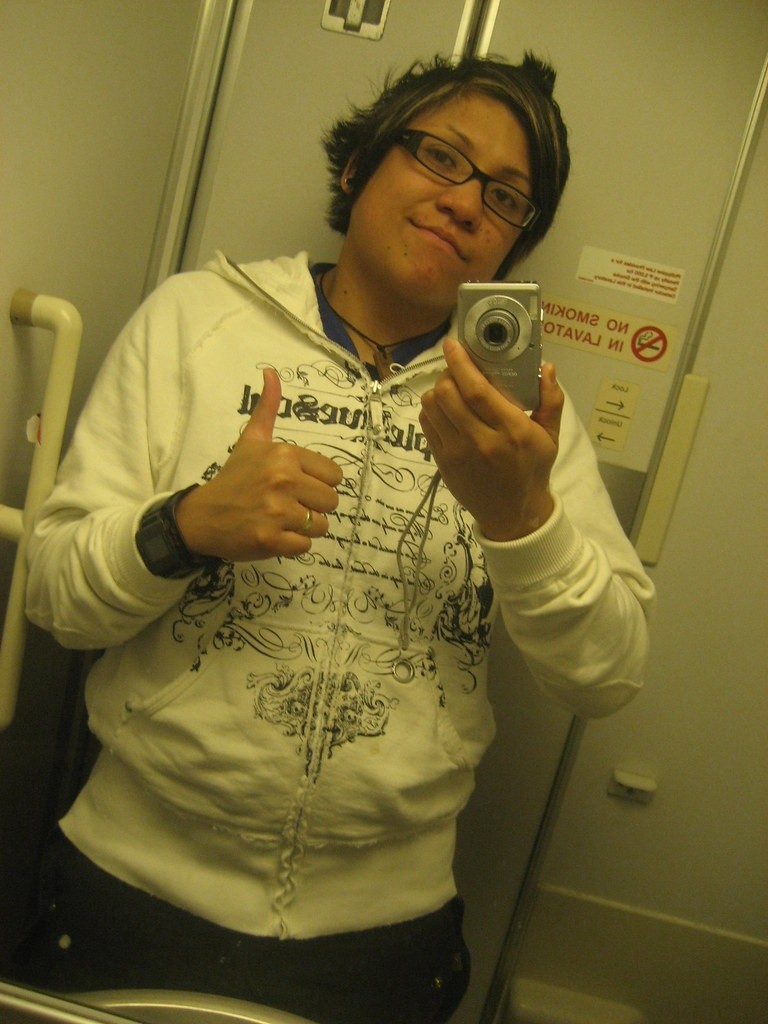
[319,271,452,382]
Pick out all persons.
[23,39,658,1024]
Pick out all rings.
[296,508,313,532]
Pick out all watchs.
[135,482,219,579]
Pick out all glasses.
[393,127,541,232]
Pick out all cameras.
[456,282,542,414]
